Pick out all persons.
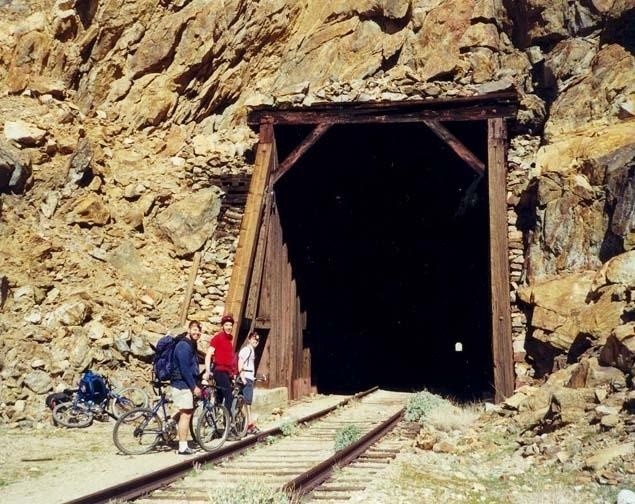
[203,314,242,441]
[169,319,205,454]
[237,332,264,436]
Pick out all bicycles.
[113,369,248,456]
[51,369,149,429]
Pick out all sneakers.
[247,425,261,434]
[222,431,241,441]
[179,448,197,454]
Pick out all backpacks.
[154,336,195,381]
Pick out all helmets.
[221,315,234,325]
